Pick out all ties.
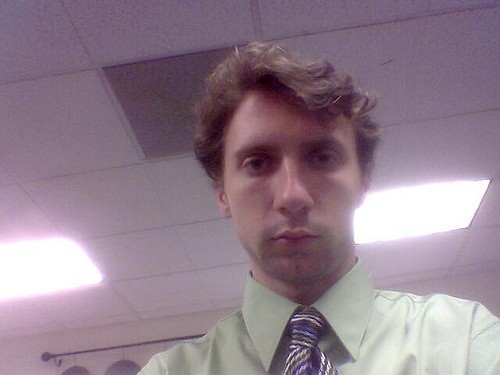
[280,308,338,375]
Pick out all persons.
[138,41,500,375]
